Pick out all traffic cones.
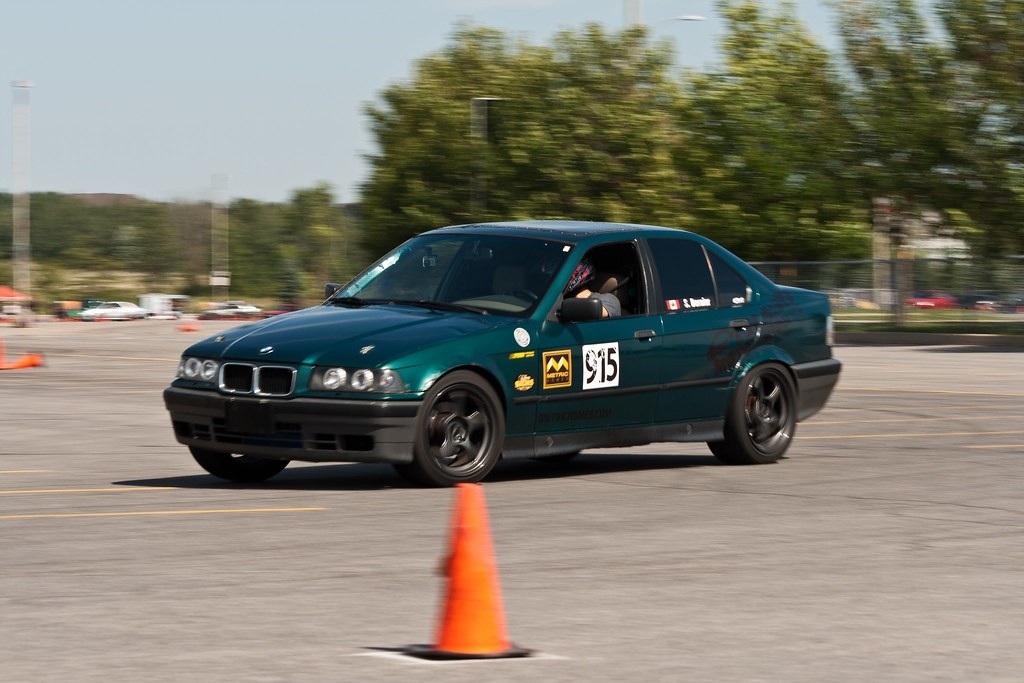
[3,348,47,368]
[400,484,546,662]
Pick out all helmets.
[544,249,595,296]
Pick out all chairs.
[491,265,529,294]
[594,271,633,315]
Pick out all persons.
[563,257,621,317]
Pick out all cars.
[161,219,843,485]
[74,300,152,320]
